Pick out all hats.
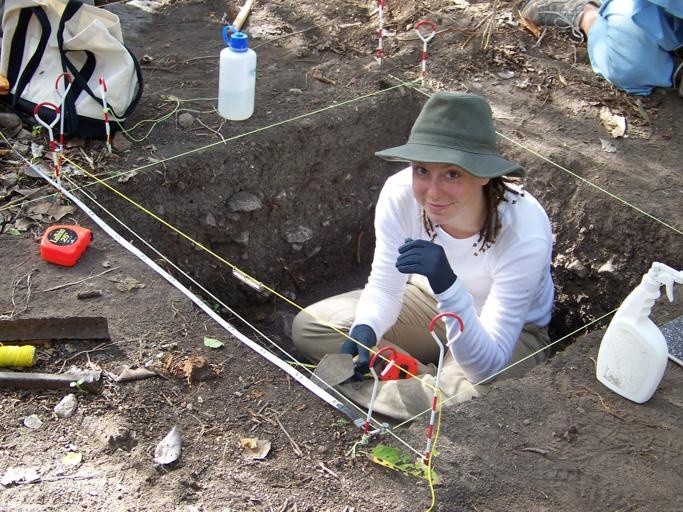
[373,89,528,179]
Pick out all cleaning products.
[595,261,683,405]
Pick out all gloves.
[339,323,377,386]
[395,236,457,296]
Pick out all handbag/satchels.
[0,0,144,140]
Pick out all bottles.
[218,25,257,122]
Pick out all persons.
[290,93,553,421]
[520,0,682,99]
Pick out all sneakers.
[521,0,602,30]
